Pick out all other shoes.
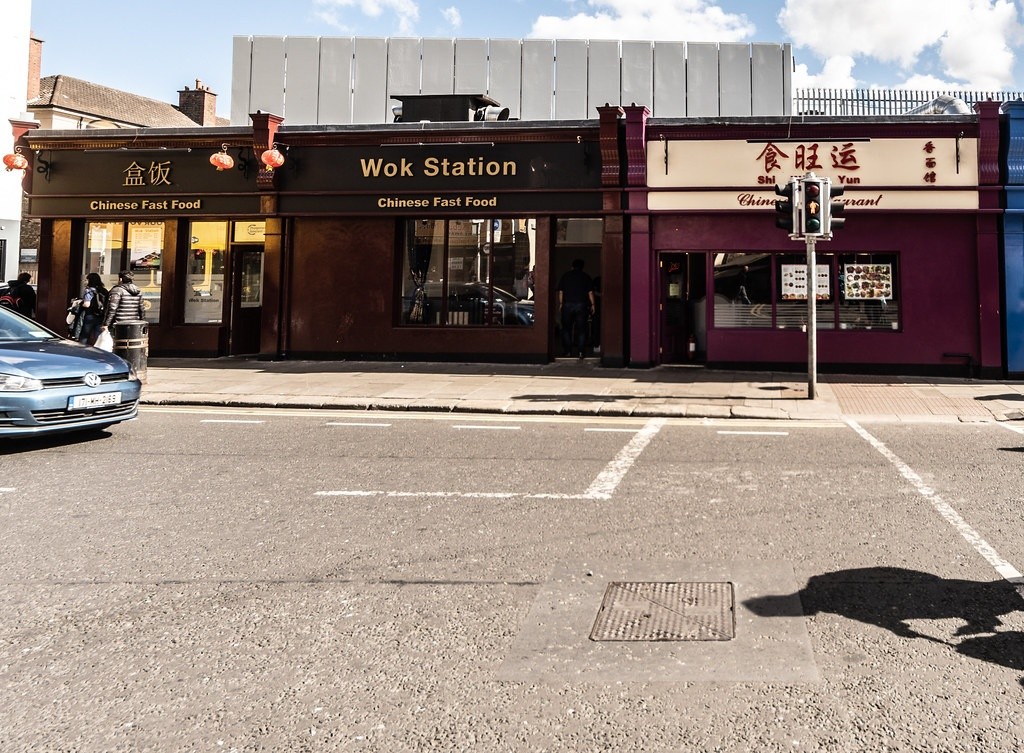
[560,352,571,356]
[579,353,584,358]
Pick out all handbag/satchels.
[94,330,113,352]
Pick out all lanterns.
[261,150,285,172]
[3,153,28,171]
[210,152,234,171]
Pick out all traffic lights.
[824,177,846,237]
[800,180,821,236]
[775,182,794,237]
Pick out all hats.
[121,270,135,280]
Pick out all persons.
[557,258,595,358]
[71,273,108,345]
[7,273,36,318]
[101,270,145,336]
[592,275,600,353]
[732,265,751,304]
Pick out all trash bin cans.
[113,320,149,386]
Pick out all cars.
[138,292,223,323]
[422,281,535,325]
[0,304,142,437]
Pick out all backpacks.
[90,287,105,316]
[4,283,26,297]
